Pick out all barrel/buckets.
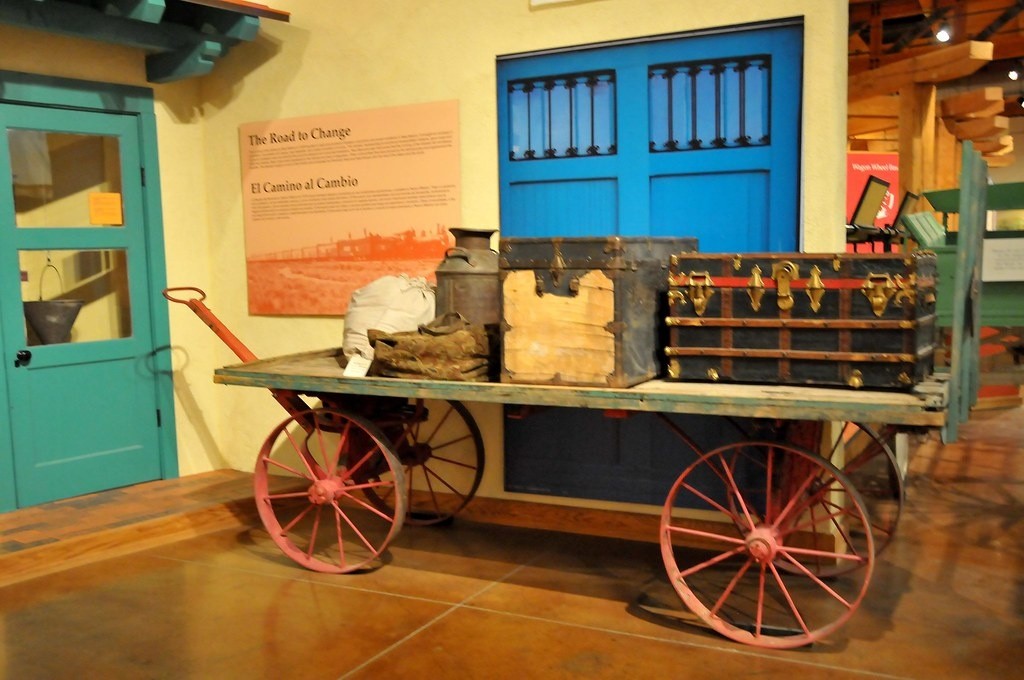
[22,265,85,346]
[435,228,499,333]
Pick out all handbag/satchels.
[368,309,491,385]
